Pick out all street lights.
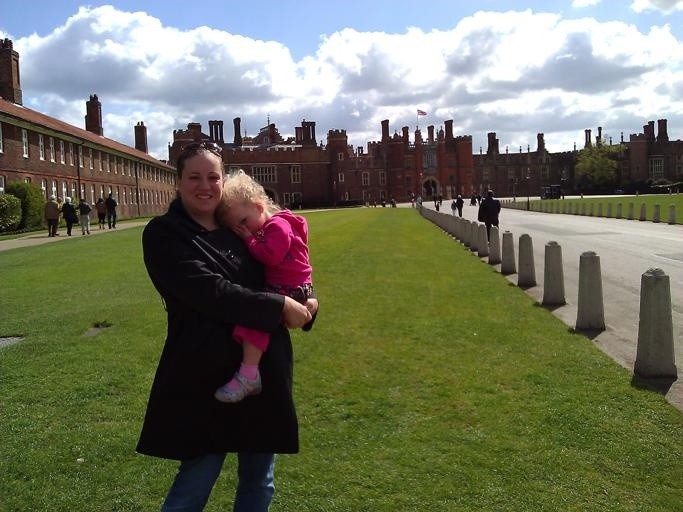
[418,172,423,197]
[523,167,531,210]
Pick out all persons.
[455,195,464,217]
[416,193,421,206]
[75,199,92,236]
[450,201,457,216]
[130,140,320,512]
[58,197,74,236]
[43,195,61,237]
[437,194,442,205]
[432,194,436,207]
[380,200,387,207]
[104,194,117,229]
[211,167,314,406]
[477,189,501,247]
[468,193,476,206]
[95,199,105,230]
[373,200,377,208]
[475,193,481,206]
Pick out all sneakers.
[48,225,116,237]
[214,368,262,403]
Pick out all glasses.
[179,142,224,162]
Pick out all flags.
[417,110,426,115]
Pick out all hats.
[48,194,71,203]
